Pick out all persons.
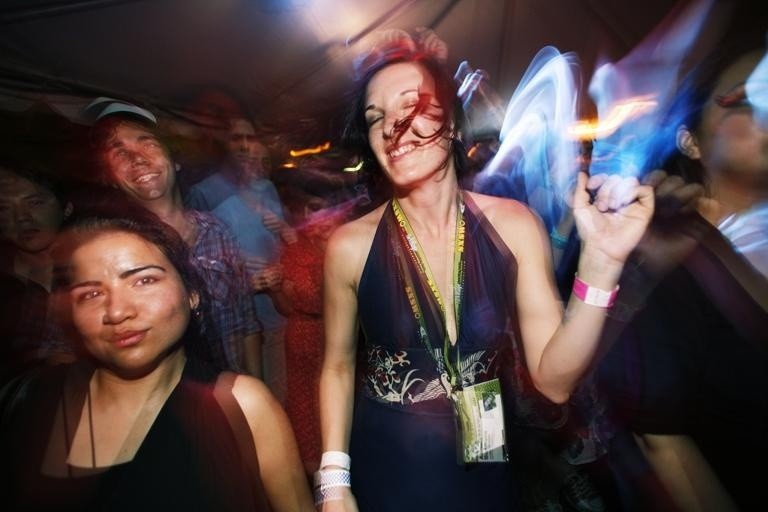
[0,29,768,512]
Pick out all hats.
[90,102,159,130]
